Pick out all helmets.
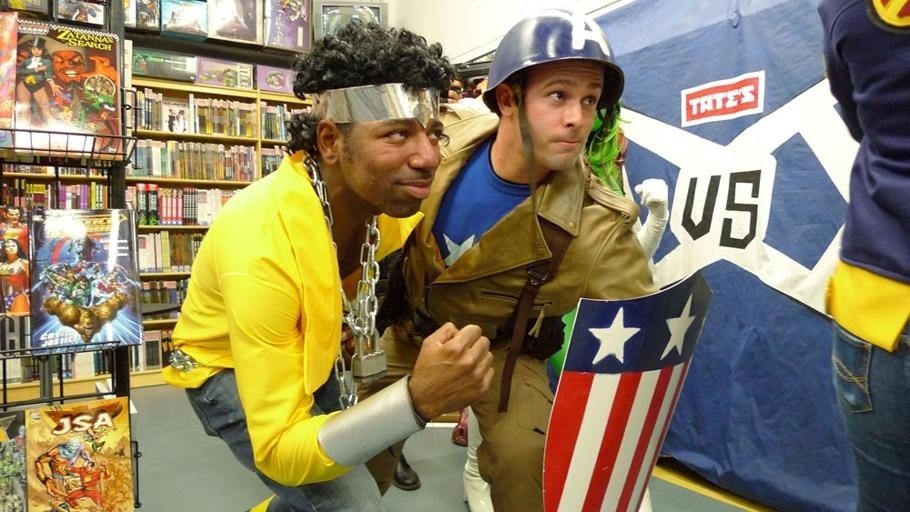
[481,8,625,110]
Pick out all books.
[143,330,173,370]
[1,0,131,160]
[124,2,381,52]
[142,281,188,320]
[130,138,290,182]
[132,45,299,97]
[136,184,239,226]
[138,232,207,274]
[134,88,312,142]
[1,164,143,511]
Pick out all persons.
[814,0,909,510]
[161,18,497,511]
[355,6,668,511]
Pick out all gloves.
[634,178,670,218]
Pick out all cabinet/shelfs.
[1,5,309,405]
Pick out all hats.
[27,36,47,50]
